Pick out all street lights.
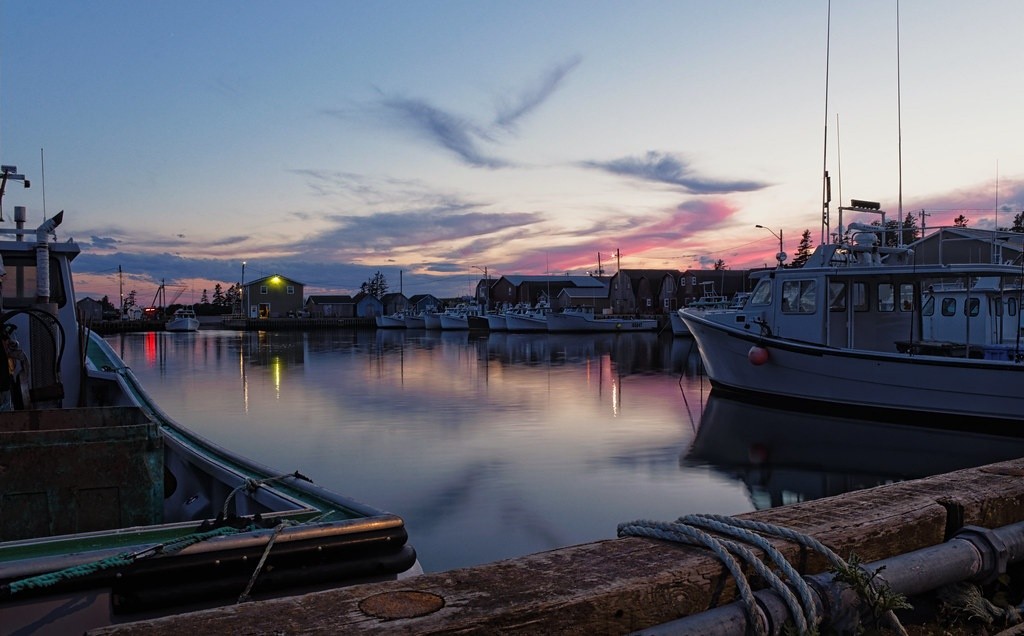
[755,224,783,266]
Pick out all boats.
[546,303,659,333]
[375,287,596,333]
[1,164,415,623]
[164,304,200,333]
[678,2,1024,434]
[669,281,752,336]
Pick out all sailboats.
[221,291,250,331]
